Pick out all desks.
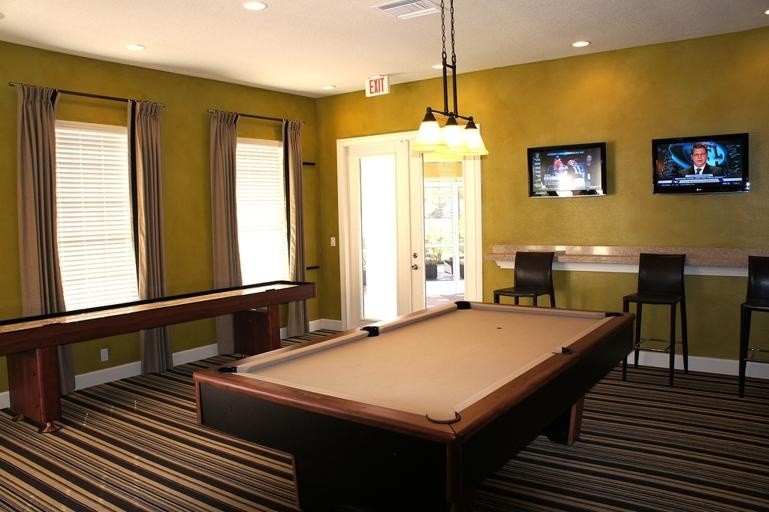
[0,280,315,434]
[192,299,637,512]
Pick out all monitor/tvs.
[527,141,608,199]
[650,132,751,196]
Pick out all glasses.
[692,153,706,157]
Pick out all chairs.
[737,256,769,398]
[622,253,688,387]
[494,253,556,309]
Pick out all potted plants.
[424,253,438,280]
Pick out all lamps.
[412,0,490,157]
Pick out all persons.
[548,154,594,186]
[678,142,725,177]
